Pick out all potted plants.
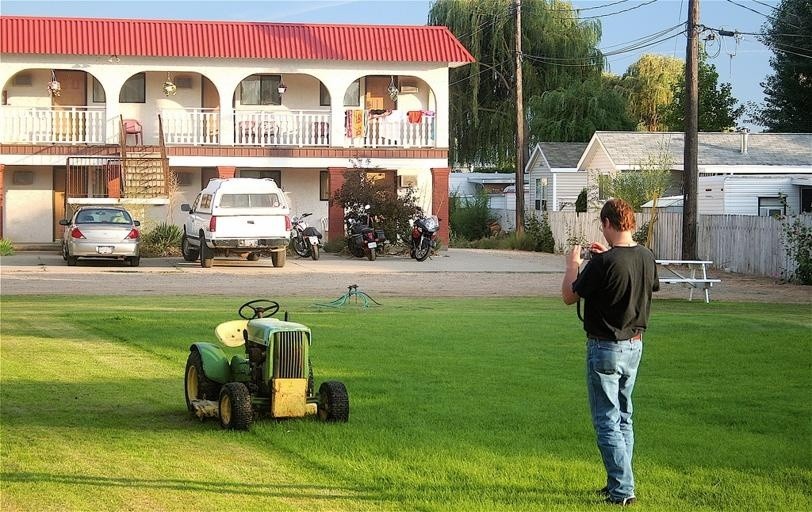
[276,75,287,94]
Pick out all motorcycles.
[410,206,442,260]
[287,212,324,260]
[344,204,384,260]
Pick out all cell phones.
[580,246,597,261]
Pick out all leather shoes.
[603,497,635,504]
[594,487,610,495]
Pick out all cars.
[59,205,140,267]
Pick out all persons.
[559,197,662,508]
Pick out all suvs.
[180,176,292,268]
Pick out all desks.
[655,259,714,279]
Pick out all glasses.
[599,221,605,232]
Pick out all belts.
[628,335,643,339]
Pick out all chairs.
[119,113,143,146]
[238,119,281,143]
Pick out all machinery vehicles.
[184,299,351,431]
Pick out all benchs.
[659,278,722,304]
